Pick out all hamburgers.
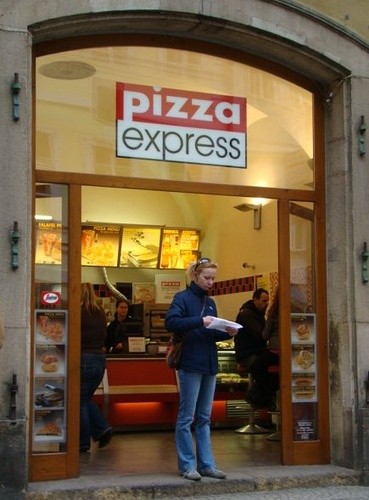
[294,378,315,399]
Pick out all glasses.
[194,257,210,271]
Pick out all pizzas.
[37,316,65,342]
[35,422,63,437]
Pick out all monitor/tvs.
[117,322,144,338]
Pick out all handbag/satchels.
[167,330,191,368]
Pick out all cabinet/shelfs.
[133,303,173,354]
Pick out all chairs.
[234,349,280,441]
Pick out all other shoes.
[182,469,226,479]
[79,442,90,452]
[98,427,118,448]
[247,391,275,410]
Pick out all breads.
[296,324,310,340]
[295,350,316,371]
[39,352,60,373]
